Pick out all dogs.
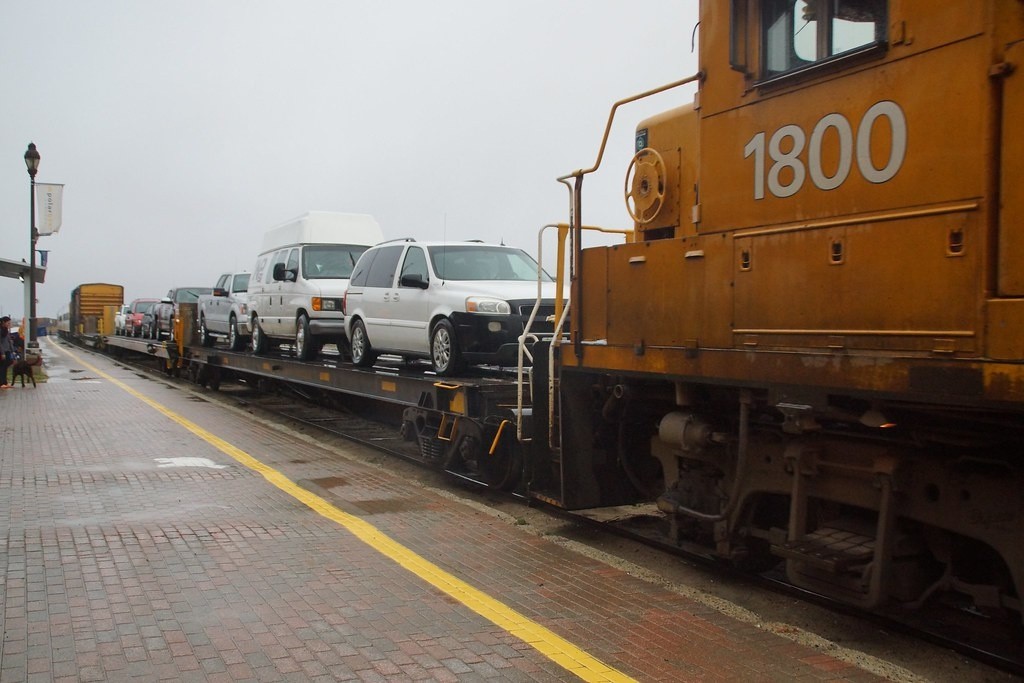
[11,356,40,388]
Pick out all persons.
[0,316,16,388]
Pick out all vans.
[245,210,386,359]
[124,298,162,337]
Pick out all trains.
[56,1,1023,672]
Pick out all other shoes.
[0,385,15,388]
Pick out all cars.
[196,271,253,351]
[343,238,572,379]
[115,305,130,335]
[156,287,214,341]
[141,299,174,341]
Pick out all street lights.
[23,142,41,366]
